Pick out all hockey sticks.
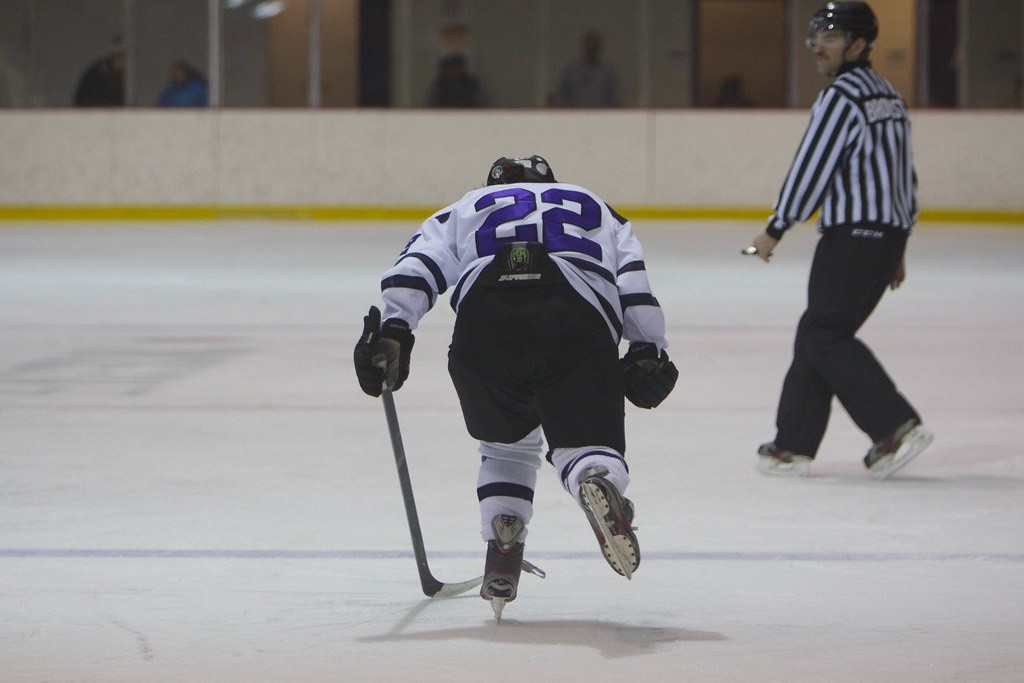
[367,305,484,598]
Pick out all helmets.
[487,155,554,185]
[804,0,878,51]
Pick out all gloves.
[353,305,415,398]
[620,341,679,409]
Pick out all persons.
[753,0,924,479]
[425,21,492,107]
[72,34,126,108]
[544,31,619,108]
[352,154,680,601]
[157,56,207,108]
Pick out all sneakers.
[577,465,641,580]
[864,417,934,480]
[758,441,813,478]
[480,513,545,625]
